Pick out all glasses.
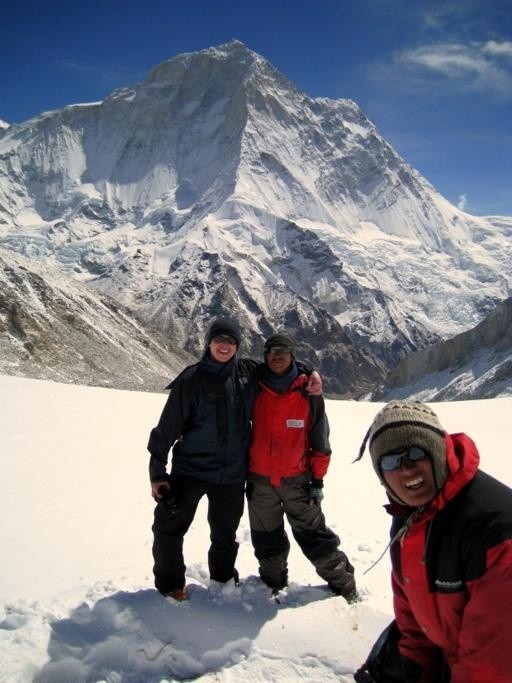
[212,335,239,347]
[267,348,290,357]
[381,446,426,471]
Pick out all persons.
[244,334,361,605]
[354,399,511,682]
[149,317,323,602]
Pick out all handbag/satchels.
[354,620,452,683]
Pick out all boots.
[167,586,183,602]
[328,583,360,604]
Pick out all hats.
[264,333,296,362]
[207,318,242,352]
[369,399,449,506]
